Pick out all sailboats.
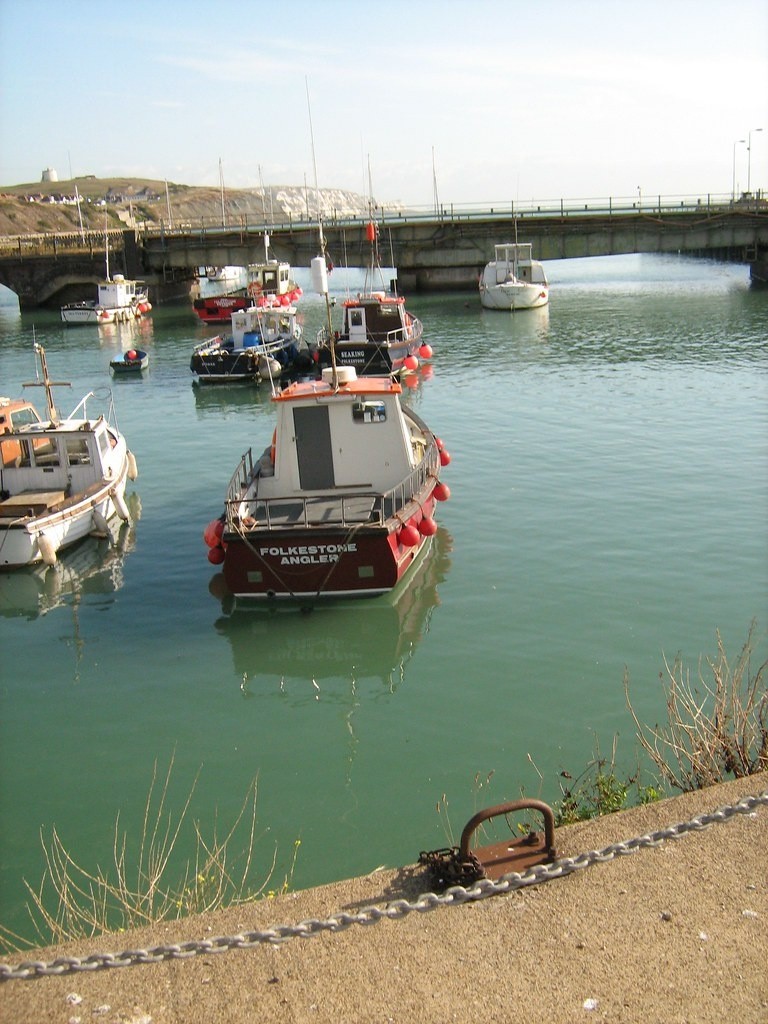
[313,152,423,379]
[191,164,303,325]
[60,194,152,324]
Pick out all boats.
[189,293,303,383]
[202,366,450,600]
[110,348,150,372]
[478,212,549,310]
[0,397,50,465]
[0,343,138,572]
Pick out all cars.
[94,200,106,206]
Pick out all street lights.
[732,140,746,204]
[747,128,763,193]
